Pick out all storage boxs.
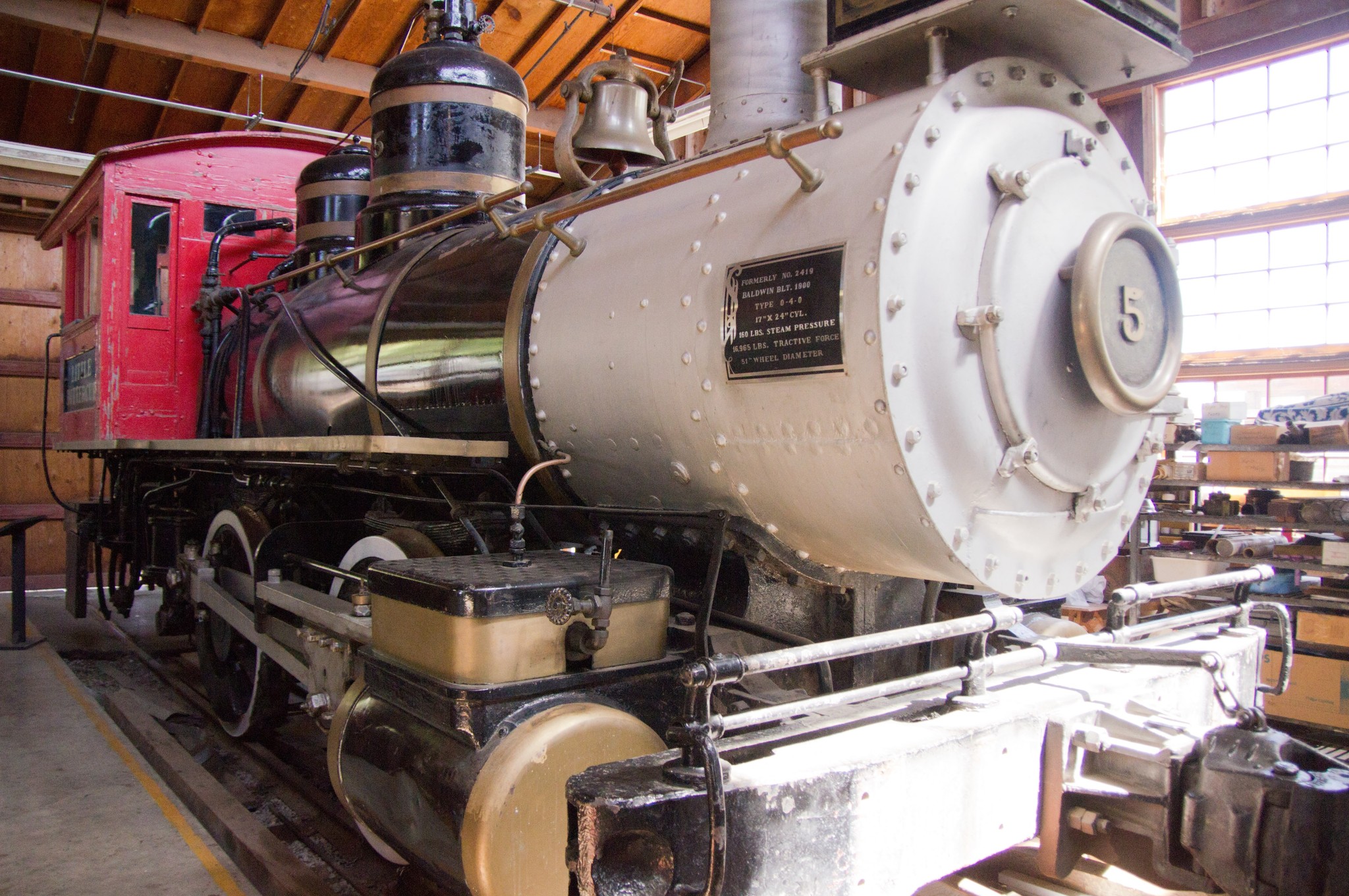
[1303,419,1349,444]
[1171,461,1207,482]
[1259,609,1349,730]
[1149,554,1229,585]
[1208,424,1288,483]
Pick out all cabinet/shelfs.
[1128,342,1349,734]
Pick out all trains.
[38,1,1295,896]
